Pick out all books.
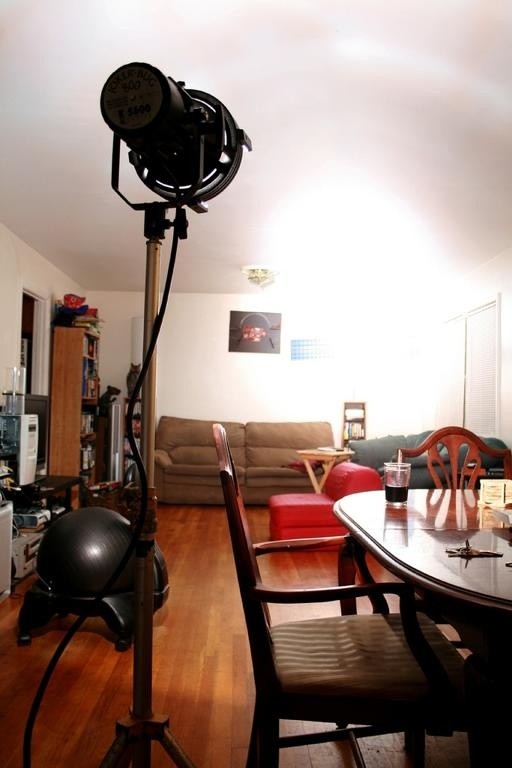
[344,407,365,462]
[78,333,98,493]
[318,445,344,451]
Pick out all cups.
[382,461,413,510]
[2,365,27,417]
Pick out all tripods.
[97,209,198,768]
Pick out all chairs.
[202,423,468,766]
[266,460,383,547]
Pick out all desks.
[297,448,355,494]
[13,474,81,531]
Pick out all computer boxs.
[0,413,39,487]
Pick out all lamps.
[95,53,255,764]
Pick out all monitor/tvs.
[3,391,48,482]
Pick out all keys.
[446,546,503,560]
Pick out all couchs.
[348,428,511,488]
[244,422,335,504]
[154,415,246,503]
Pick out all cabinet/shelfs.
[46,321,102,507]
